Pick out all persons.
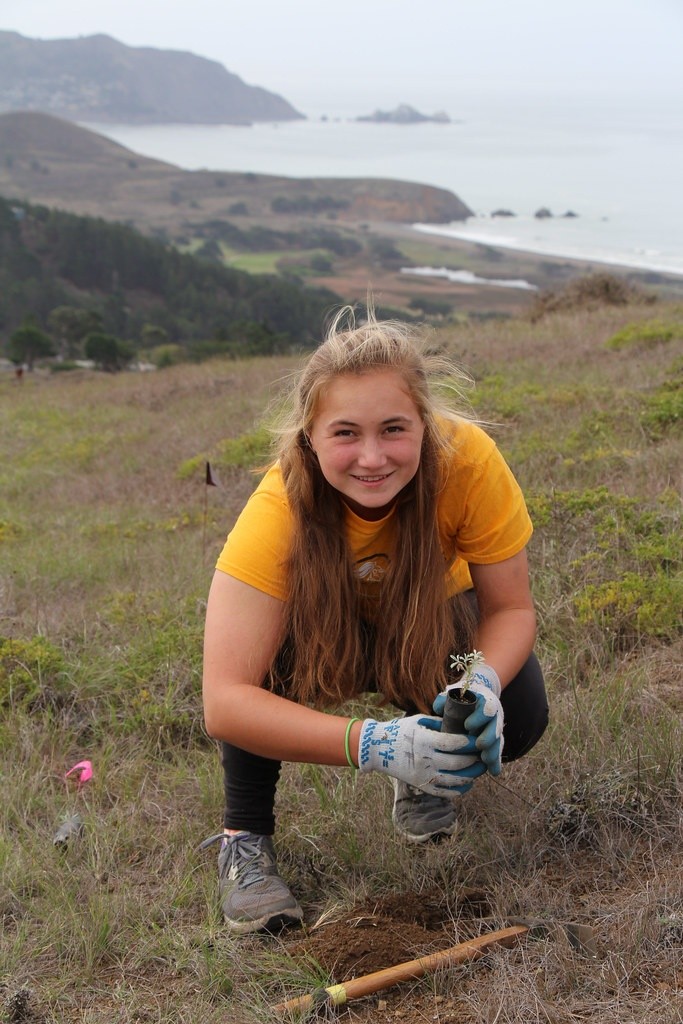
[201,292,550,935]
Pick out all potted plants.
[441,648,486,734]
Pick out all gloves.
[433,662,504,776]
[357,713,487,799]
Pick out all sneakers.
[193,830,303,935]
[387,775,458,846]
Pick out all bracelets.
[344,717,360,770]
